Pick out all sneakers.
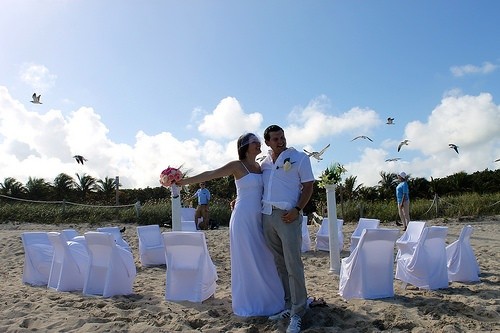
[286,314,301,333]
[269,309,291,320]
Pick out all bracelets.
[295,207,301,212]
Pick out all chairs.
[338,228,401,299]
[314,218,344,253]
[350,218,380,255]
[136,225,166,267]
[62,229,88,250]
[394,226,449,291]
[46,231,89,292]
[446,225,481,282]
[96,227,130,252]
[301,215,310,252]
[161,231,218,304]
[21,232,54,287]
[82,231,134,298]
[395,221,427,263]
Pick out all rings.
[288,220,290,222]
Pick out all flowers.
[284,161,292,171]
[159,167,184,199]
[317,161,348,189]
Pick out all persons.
[176,133,285,317]
[186,182,210,230]
[396,172,410,231]
[230,125,314,333]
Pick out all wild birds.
[30,93,44,105]
[303,117,500,163]
[73,155,88,165]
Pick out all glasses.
[200,183,205,185]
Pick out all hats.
[399,171,406,179]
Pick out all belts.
[198,204,207,205]
[272,205,278,211]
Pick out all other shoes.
[402,226,407,231]
[307,296,326,308]
